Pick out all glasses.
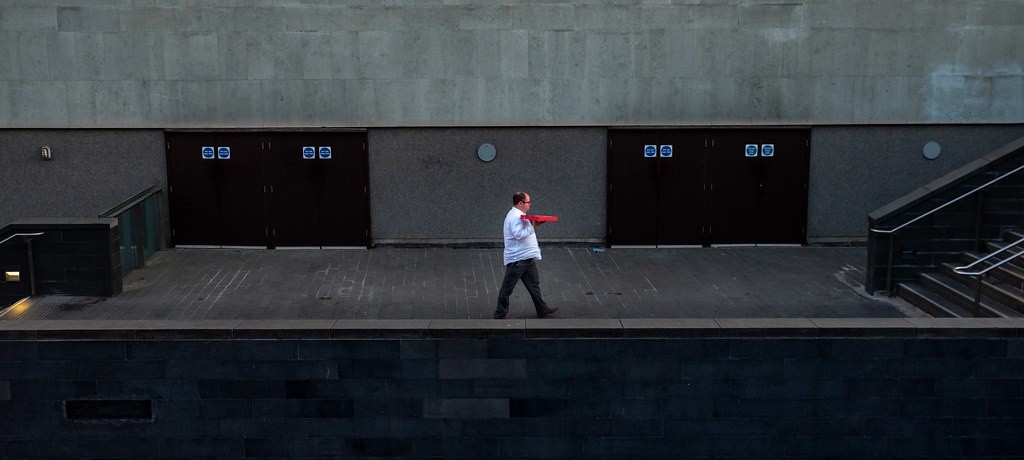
[523,201,531,204]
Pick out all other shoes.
[493,314,507,319]
[537,307,559,318]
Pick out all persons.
[494,192,560,319]
